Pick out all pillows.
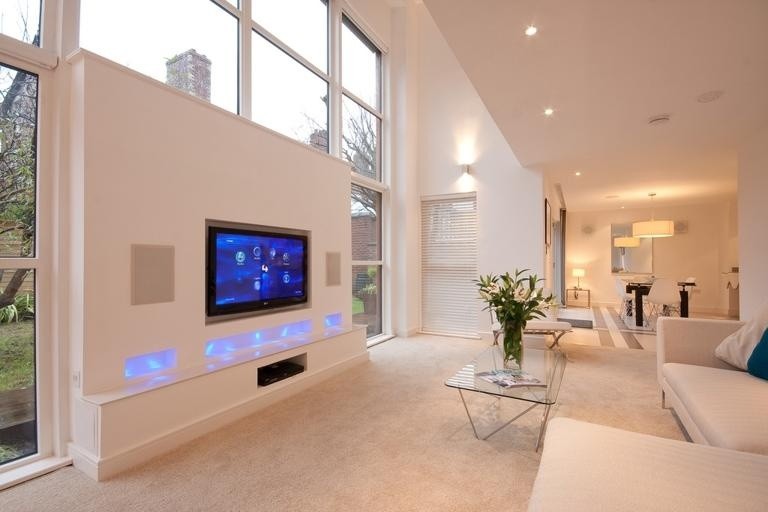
[715,314,768,380]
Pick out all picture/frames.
[545,198,551,248]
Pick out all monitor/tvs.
[208,226,307,316]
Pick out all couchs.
[527,417,768,512]
[657,317,768,456]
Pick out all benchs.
[489,321,572,364]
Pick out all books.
[491,369,547,388]
[472,372,521,389]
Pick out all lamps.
[613,237,641,247]
[573,269,585,289]
[632,194,674,238]
[460,161,473,176]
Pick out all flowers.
[472,268,561,365]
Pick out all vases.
[499,324,525,370]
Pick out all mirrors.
[610,223,653,275]
